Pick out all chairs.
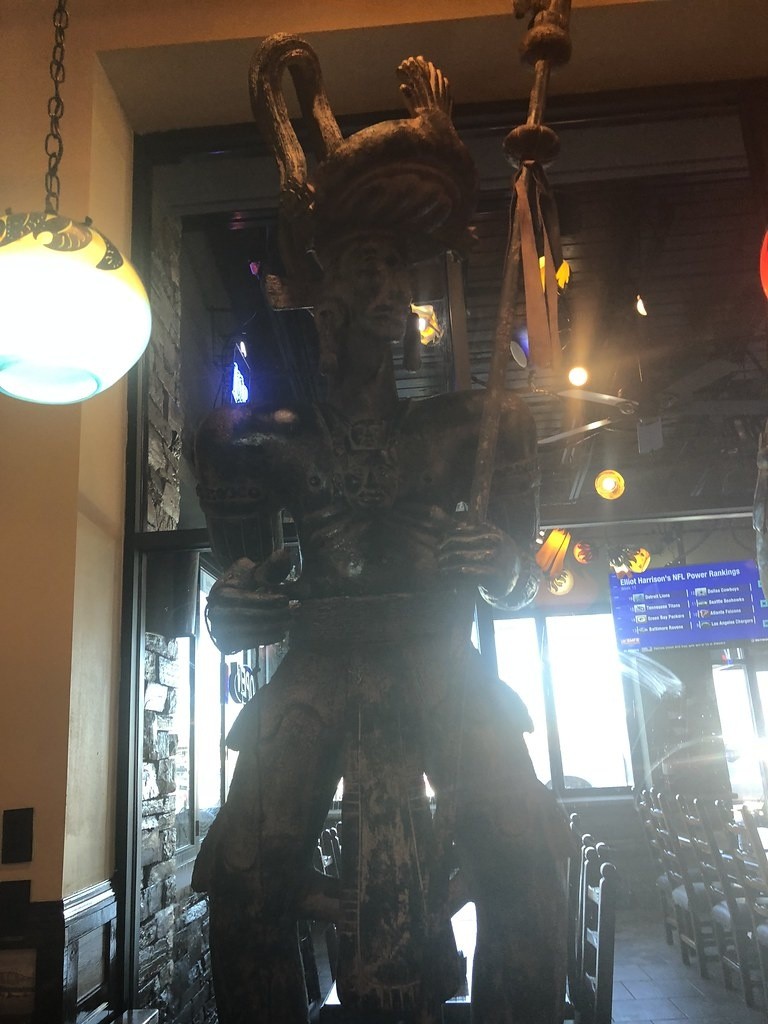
[557,806,621,1024]
[629,784,767,1007]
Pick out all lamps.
[0,3,155,410]
